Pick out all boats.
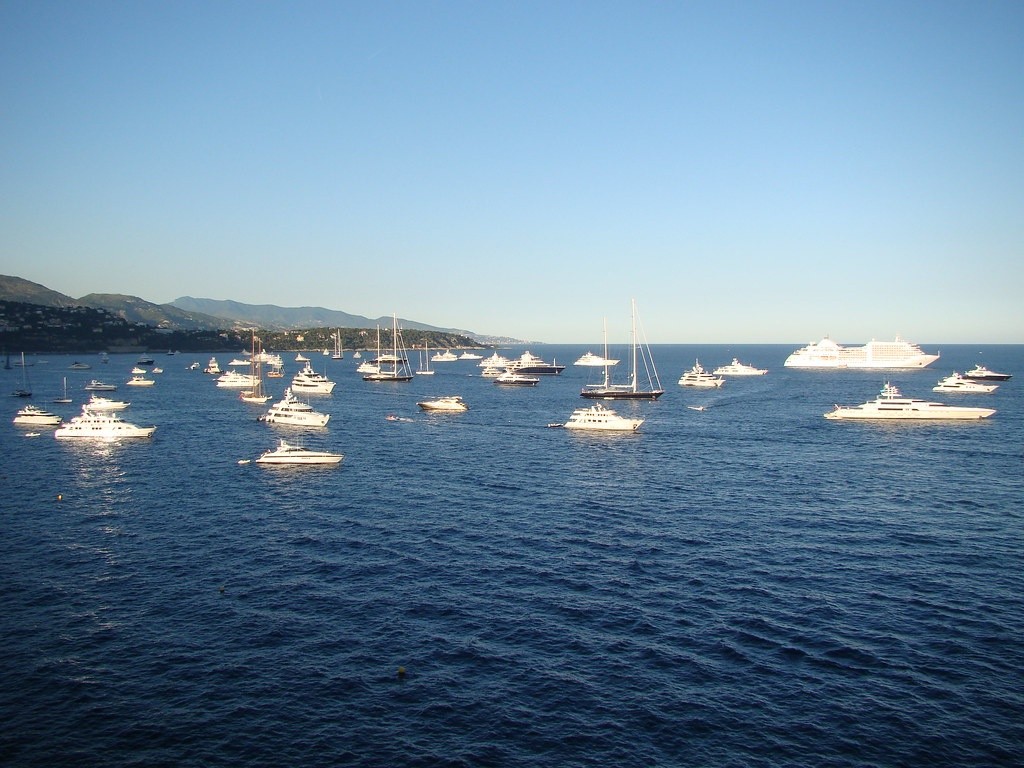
[823,380,998,419]
[784,334,940,368]
[291,361,336,394]
[86,393,132,411]
[563,403,645,431]
[55,404,158,438]
[678,357,770,386]
[12,405,63,425]
[932,369,999,392]
[264,387,330,427]
[958,364,1014,381]
[416,397,468,411]
[67,348,622,386]
[83,379,118,391]
[255,439,345,463]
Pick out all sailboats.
[330,312,434,381]
[55,377,73,402]
[11,351,33,397]
[241,328,267,403]
[579,298,666,401]
